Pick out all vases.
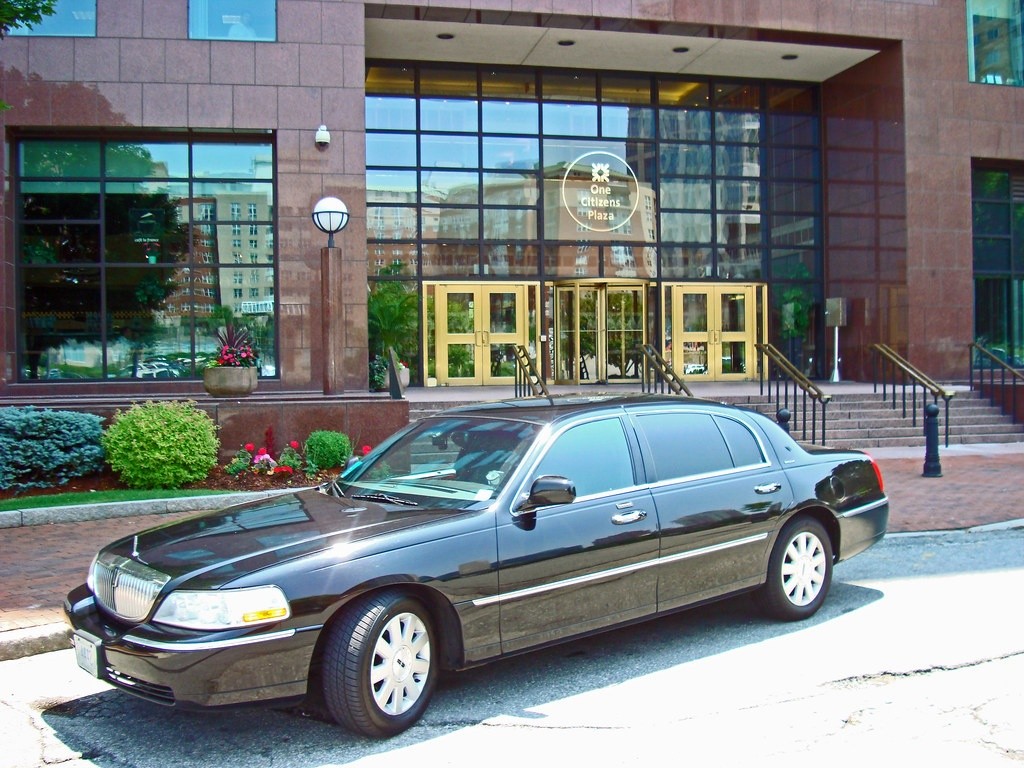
[203,365,258,397]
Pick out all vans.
[686,364,705,374]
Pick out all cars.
[24,356,215,379]
[976,348,1023,368]
[63,392,890,738]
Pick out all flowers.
[214,323,261,368]
[399,359,409,367]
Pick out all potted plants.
[369,348,410,391]
